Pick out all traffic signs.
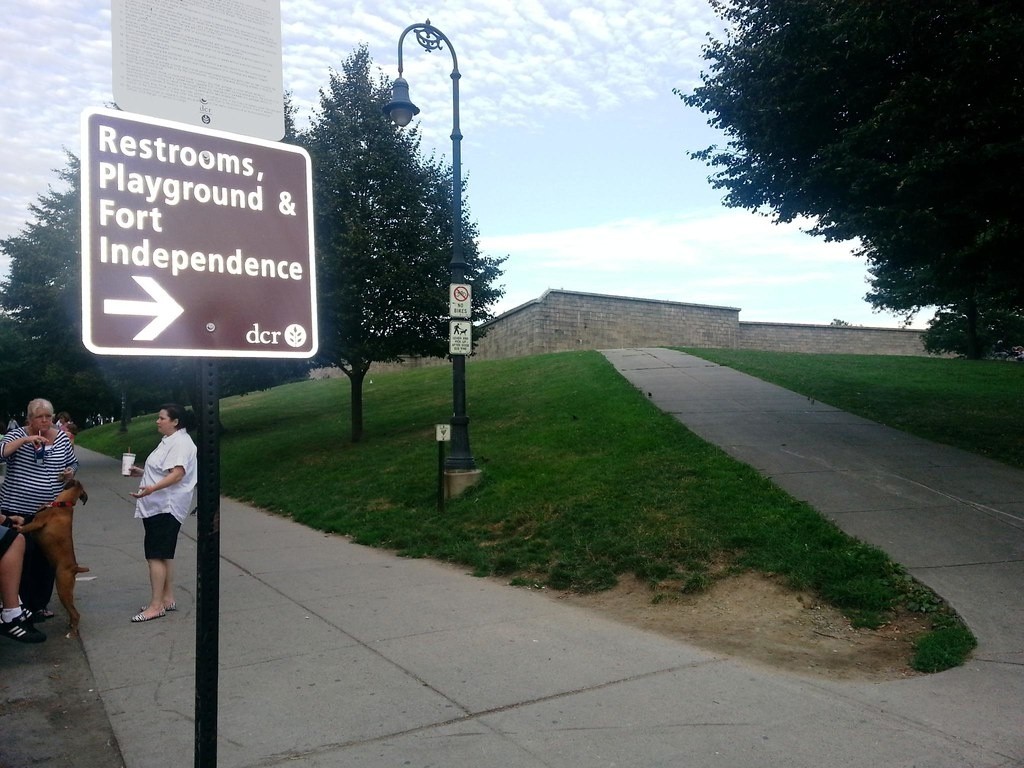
[78,109,324,359]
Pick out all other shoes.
[40,608,54,618]
[32,607,44,618]
[131,608,166,622]
[141,601,176,611]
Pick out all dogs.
[12,478,90,638]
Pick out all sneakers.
[0,610,46,643]
[0,594,45,623]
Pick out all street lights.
[381,21,482,499]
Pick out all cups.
[33,441,46,465]
[122,453,136,475]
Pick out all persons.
[55,412,78,451]
[0,515,48,644]
[130,403,198,622]
[7,417,20,432]
[0,398,78,621]
